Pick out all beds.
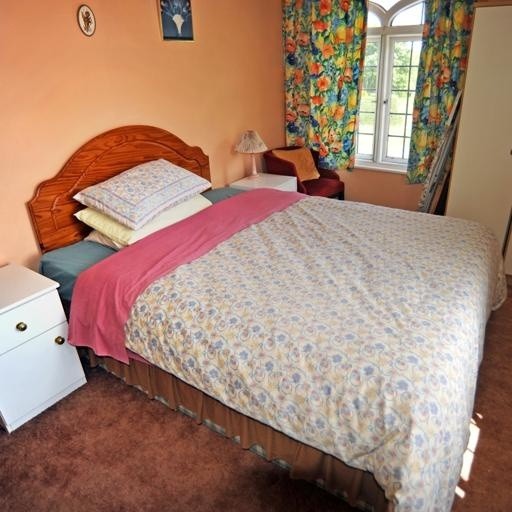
[25,124,507,512]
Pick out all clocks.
[77,3,97,37]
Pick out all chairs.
[262,145,344,200]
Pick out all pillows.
[271,147,321,182]
[73,158,213,252]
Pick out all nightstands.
[229,172,298,192]
[0,262,88,435]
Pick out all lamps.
[233,130,268,180]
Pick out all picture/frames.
[156,1,196,43]
